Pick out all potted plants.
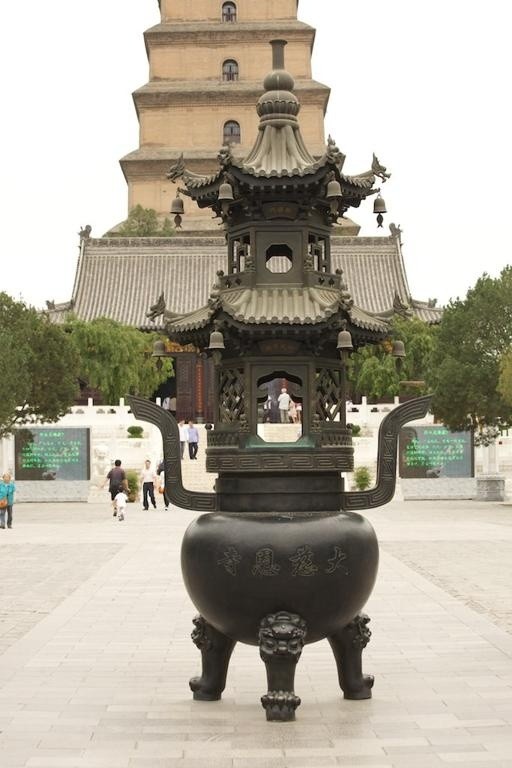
[122,471,138,502]
[127,426,144,447]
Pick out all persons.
[1,471,18,529]
[158,457,171,511]
[402,427,432,477]
[141,460,158,510]
[99,459,132,517]
[168,393,177,417]
[178,418,188,460]
[261,394,276,423]
[109,485,131,520]
[295,403,302,423]
[186,420,200,459]
[162,392,170,413]
[287,397,298,425]
[278,387,293,423]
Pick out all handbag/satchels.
[0,495,8,509]
[158,486,164,494]
